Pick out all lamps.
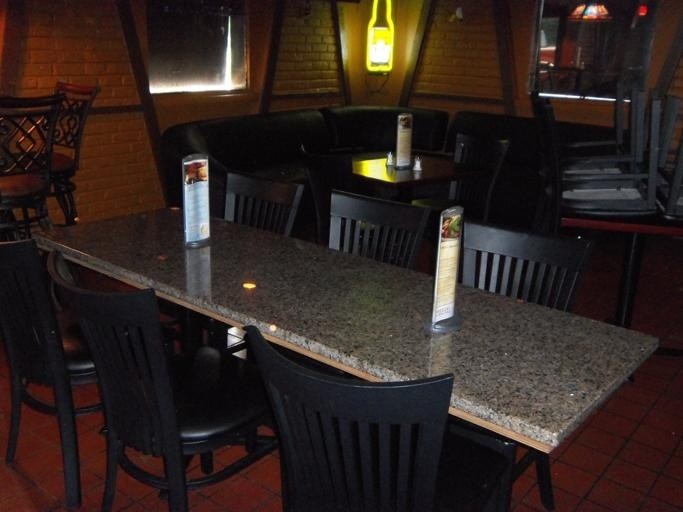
[567,0,614,23]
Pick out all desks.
[29,207,661,461]
[557,214,682,388]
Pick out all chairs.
[325,183,435,273]
[48,278,280,512]
[1,81,103,244]
[460,220,607,510]
[0,238,127,509]
[241,324,520,512]
[223,170,304,239]
[522,68,683,238]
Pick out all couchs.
[144,105,631,268]
